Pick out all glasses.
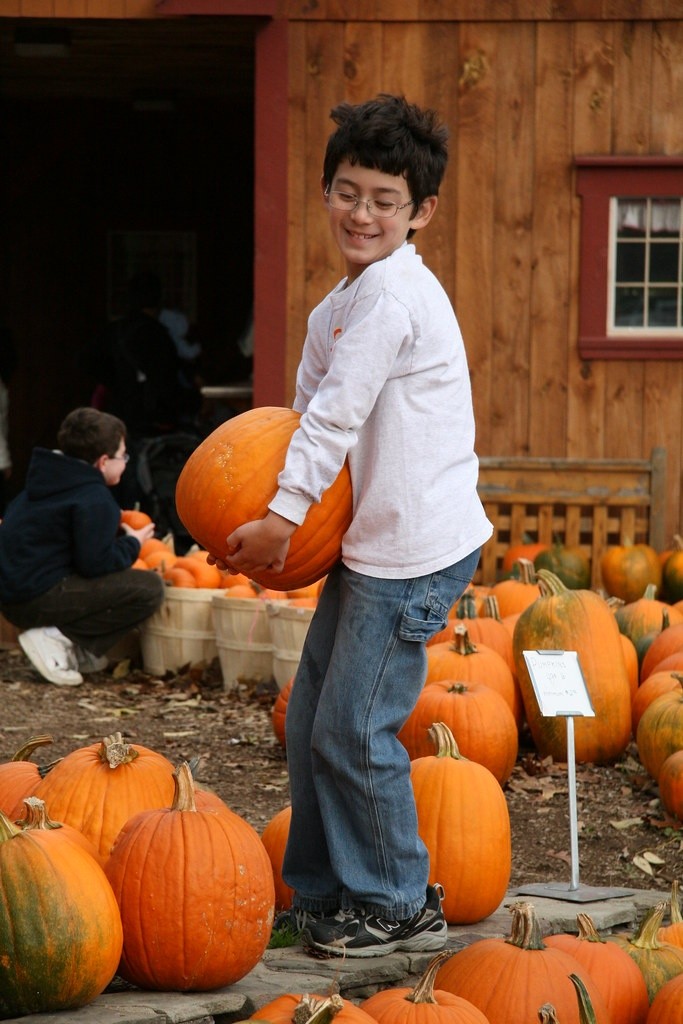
[323,184,414,218]
[112,454,130,464]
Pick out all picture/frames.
[104,228,200,326]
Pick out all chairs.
[136,432,207,548]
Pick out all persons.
[59,271,233,536]
[0,408,166,686]
[0,327,20,481]
[206,94,495,958]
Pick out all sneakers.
[73,643,111,674]
[304,881,449,957]
[270,896,340,940]
[17,626,84,685]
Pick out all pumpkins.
[177,406,354,592]
[116,501,683,822]
[0,721,683,1024]
